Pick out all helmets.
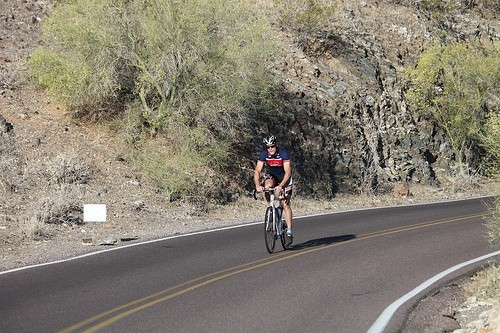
[262,135,277,148]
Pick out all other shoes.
[286,232,293,245]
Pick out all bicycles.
[253,188,290,254]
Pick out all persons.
[254,135,293,246]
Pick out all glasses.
[266,144,276,150]
